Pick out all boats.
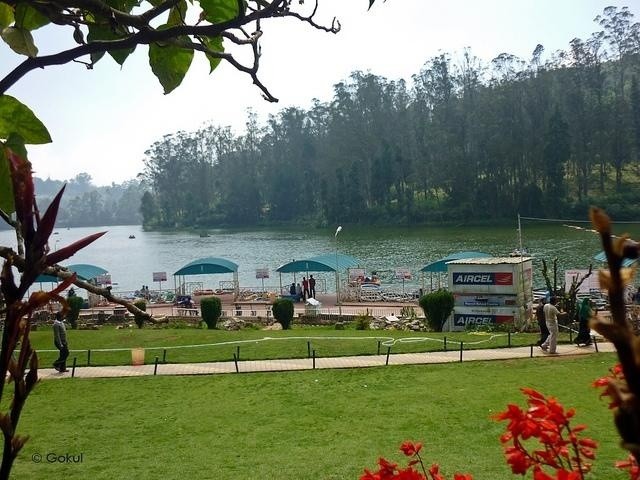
[199,231,210,237]
[510,252,530,257]
[532,287,561,304]
[129,234,136,239]
[576,294,607,311]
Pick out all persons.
[308,274,316,299]
[179,296,187,317]
[141,285,166,302]
[289,282,296,295]
[187,297,196,316]
[541,297,562,355]
[53,312,70,372]
[294,282,304,302]
[572,298,598,346]
[301,276,310,299]
[535,298,550,346]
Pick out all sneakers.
[53,362,70,372]
[574,339,581,347]
[549,351,560,354]
[537,341,548,351]
[584,343,593,346]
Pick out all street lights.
[335,226,342,323]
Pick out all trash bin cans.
[305,298,321,317]
